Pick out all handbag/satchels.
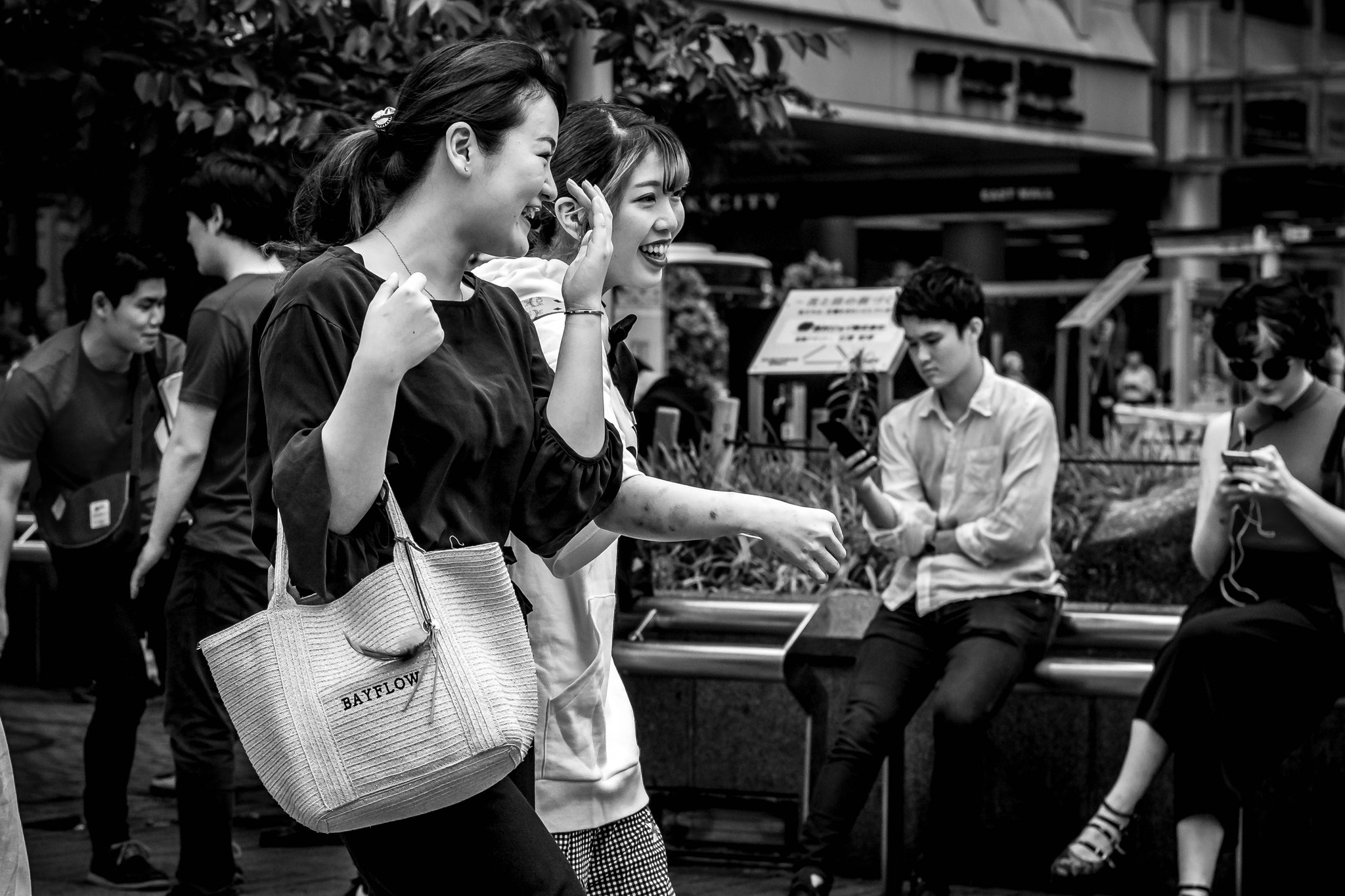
[30,471,135,566]
[195,470,540,834]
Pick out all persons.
[785,265,1066,896]
[1001,345,1171,446]
[244,41,624,896]
[468,98,848,895]
[0,250,189,888]
[1052,270,1345,896]
[130,150,366,896]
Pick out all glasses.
[1227,356,1294,380]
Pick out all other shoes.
[148,773,180,794]
[788,872,831,896]
[1048,804,1132,880]
[84,841,170,890]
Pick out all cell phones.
[1220,451,1258,484]
[817,419,878,470]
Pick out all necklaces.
[375,226,463,302]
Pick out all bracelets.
[564,309,604,317]
